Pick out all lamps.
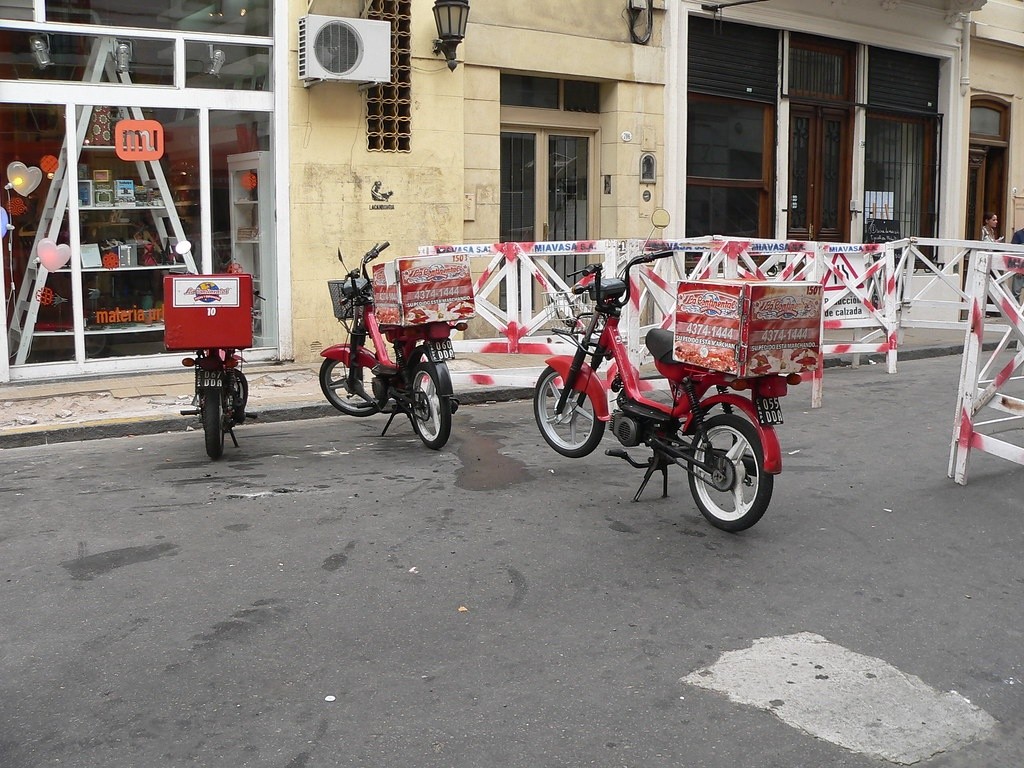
[30,34,55,71]
[431,0,470,72]
[203,44,226,80]
[112,39,134,73]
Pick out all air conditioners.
[297,13,391,92]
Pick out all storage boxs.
[78,179,95,207]
[162,274,254,350]
[672,277,821,378]
[117,238,145,268]
[372,252,476,327]
[93,169,113,182]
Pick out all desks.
[187,53,269,90]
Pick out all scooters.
[165,240,266,459]
[534,208,802,533]
[319,240,467,452]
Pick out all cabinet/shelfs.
[226,150,274,347]
[7,145,199,365]
